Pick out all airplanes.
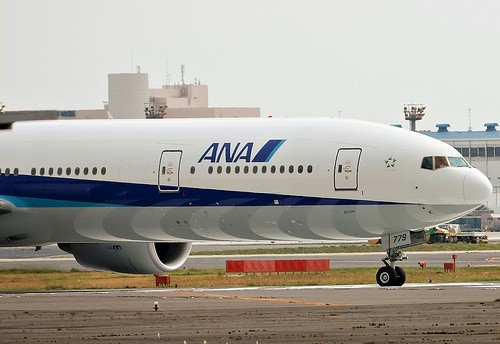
[1,119,493,288]
